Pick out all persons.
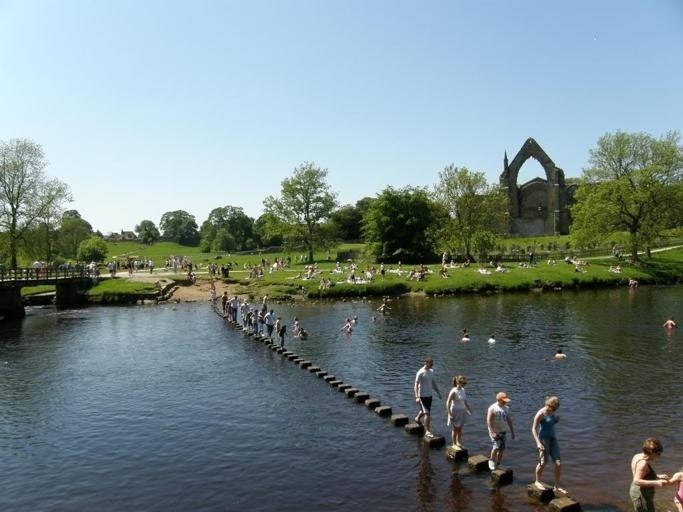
[297,262,373,289]
[414,357,443,439]
[554,349,566,358]
[349,315,358,324]
[462,328,467,335]
[486,391,515,471]
[446,375,472,451]
[166,255,195,286]
[532,395,569,495]
[528,250,588,273]
[609,245,633,273]
[381,262,385,278]
[341,318,351,333]
[371,316,376,321]
[250,256,291,278]
[661,315,677,328]
[376,302,392,313]
[630,437,669,512]
[208,262,229,278]
[487,334,495,344]
[629,280,638,288]
[409,252,470,282]
[461,333,470,341]
[33,255,153,281]
[489,257,505,272]
[669,467,682,512]
[208,289,307,347]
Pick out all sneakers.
[414,418,422,425]
[453,444,464,450]
[425,432,434,437]
[488,460,504,470]
[552,488,568,496]
[535,481,546,491]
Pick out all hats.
[496,392,511,402]
